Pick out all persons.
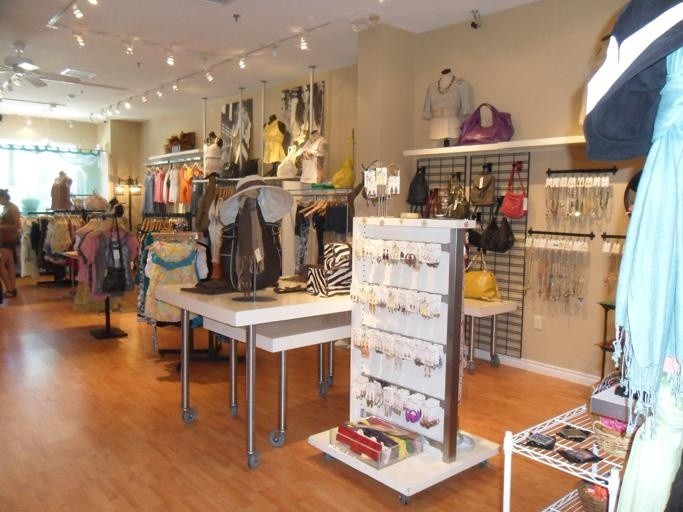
[421,67,471,142]
[0,188,20,298]
[105,195,124,218]
[282,83,324,147]
[50,170,73,210]
[294,130,331,183]
[202,130,224,176]
[261,114,286,165]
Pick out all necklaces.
[521,176,618,308]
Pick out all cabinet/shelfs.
[595,299,628,379]
[501,398,637,512]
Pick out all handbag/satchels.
[463,271,499,303]
[305,267,351,297]
[220,222,282,292]
[502,192,527,219]
[322,241,353,269]
[467,227,481,247]
[444,188,469,220]
[481,219,516,253]
[102,268,126,294]
[457,103,513,146]
[331,163,354,189]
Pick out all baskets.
[590,415,642,461]
[576,479,610,512]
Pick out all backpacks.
[470,165,496,207]
[405,167,429,206]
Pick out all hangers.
[141,212,183,232]
[215,185,236,200]
[146,158,203,174]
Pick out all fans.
[1,41,49,92]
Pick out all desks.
[460,296,517,374]
[197,315,353,449]
[159,277,354,467]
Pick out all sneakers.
[3,289,17,297]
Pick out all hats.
[581,0,683,162]
[218,175,293,228]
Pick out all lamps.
[73,0,310,124]
